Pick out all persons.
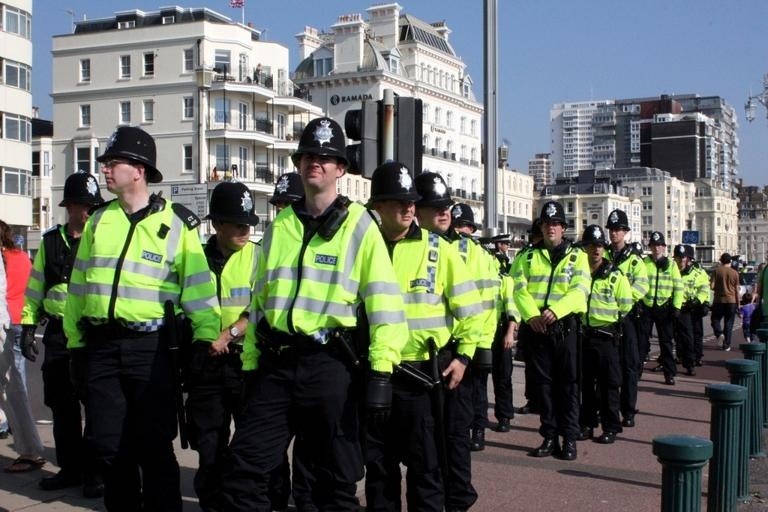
[251,63,263,85]
[0,256,11,435]
[215,116,412,512]
[60,125,223,512]
[0,218,46,475]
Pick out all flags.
[228,0,244,9]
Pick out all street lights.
[744,72,767,127]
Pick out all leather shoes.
[530,436,559,459]
[558,437,580,461]
[495,416,512,433]
[470,427,487,451]
[577,426,596,440]
[651,355,702,386]
[597,431,617,445]
[620,411,637,427]
[40,466,105,499]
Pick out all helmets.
[267,171,305,204]
[449,203,479,233]
[94,126,163,184]
[362,161,424,210]
[57,172,108,207]
[671,244,691,259]
[414,171,453,206]
[288,116,351,167]
[199,180,260,227]
[526,199,632,245]
[649,231,667,247]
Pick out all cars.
[693,256,761,311]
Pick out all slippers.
[3,449,48,475]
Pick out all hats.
[491,233,511,243]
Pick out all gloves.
[18,323,40,363]
[361,371,393,427]
